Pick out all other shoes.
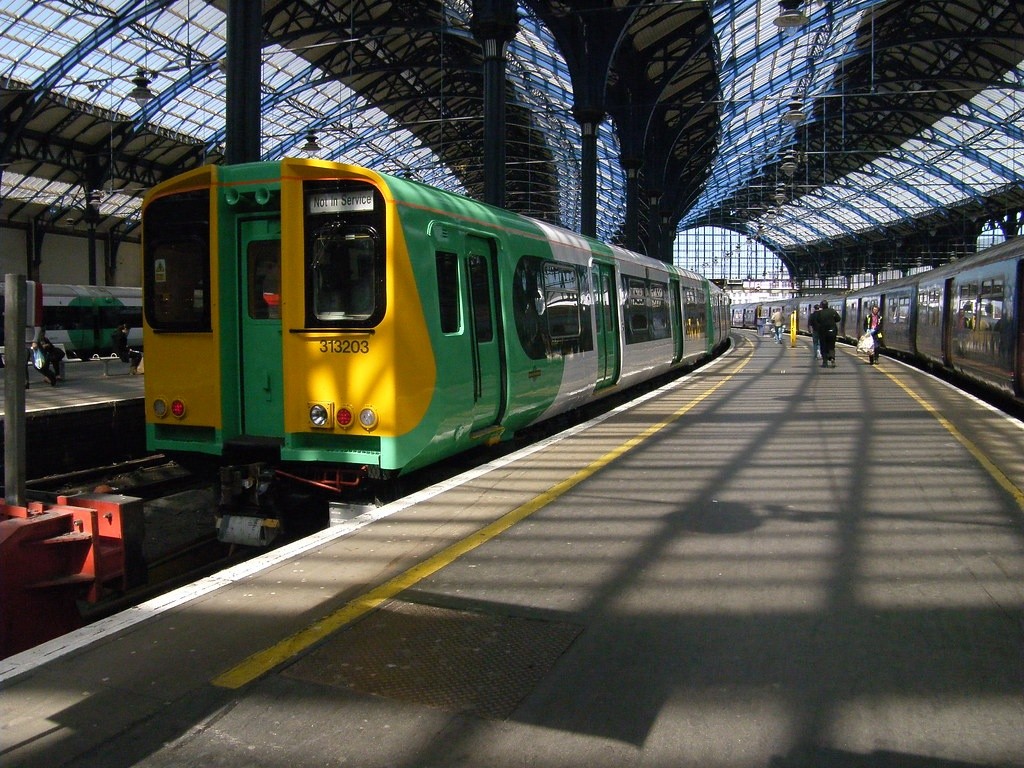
[875,360,879,364]
[822,363,827,367]
[831,359,835,367]
[50,372,55,386]
[870,361,873,364]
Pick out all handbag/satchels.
[137,357,144,374]
[49,344,65,362]
[855,331,874,355]
[33,347,45,370]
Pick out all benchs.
[27,358,82,382]
[89,353,122,379]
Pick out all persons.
[112,323,142,376]
[808,305,823,360]
[39,327,64,384]
[953,310,1013,360]
[864,306,883,365]
[24,326,57,389]
[812,300,841,367]
[770,308,784,344]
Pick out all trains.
[0,280,144,367]
[140,158,731,493]
[732,235,1024,407]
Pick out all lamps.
[772,188,787,205]
[127,72,157,107]
[766,210,776,224]
[301,131,321,157]
[779,154,798,176]
[784,97,805,127]
[799,221,959,276]
[773,0,810,38]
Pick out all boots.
[130,365,143,375]
